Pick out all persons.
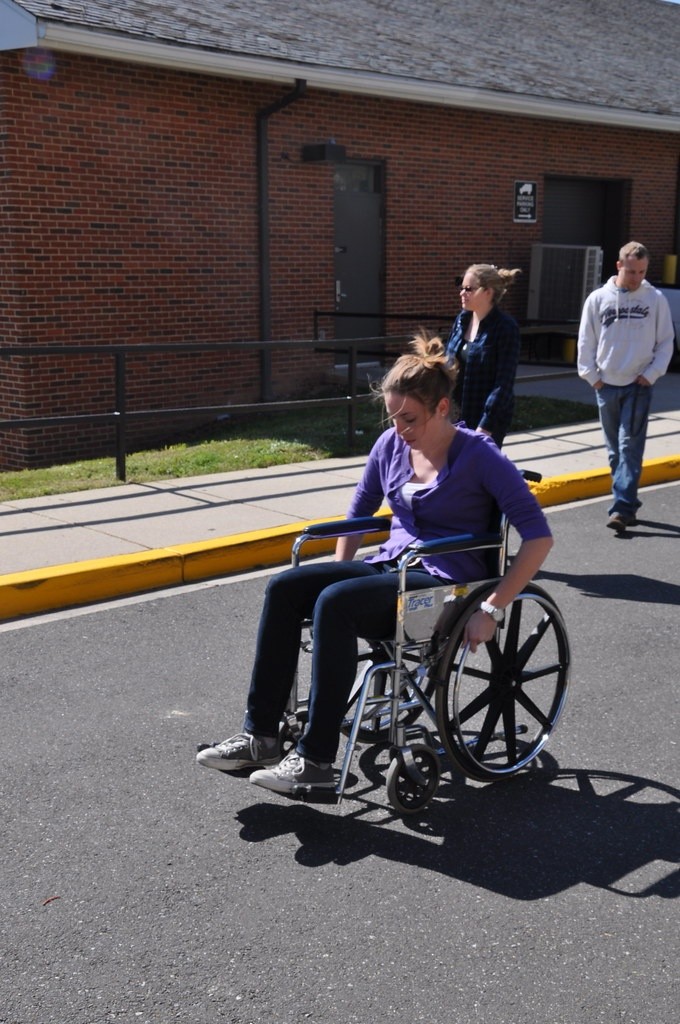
[195,324,556,793]
[444,263,525,454]
[576,241,675,533]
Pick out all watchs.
[480,601,505,622]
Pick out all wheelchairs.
[196,469,574,810]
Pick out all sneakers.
[195,731,281,770]
[249,749,336,793]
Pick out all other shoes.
[606,511,628,532]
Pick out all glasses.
[459,284,480,292]
